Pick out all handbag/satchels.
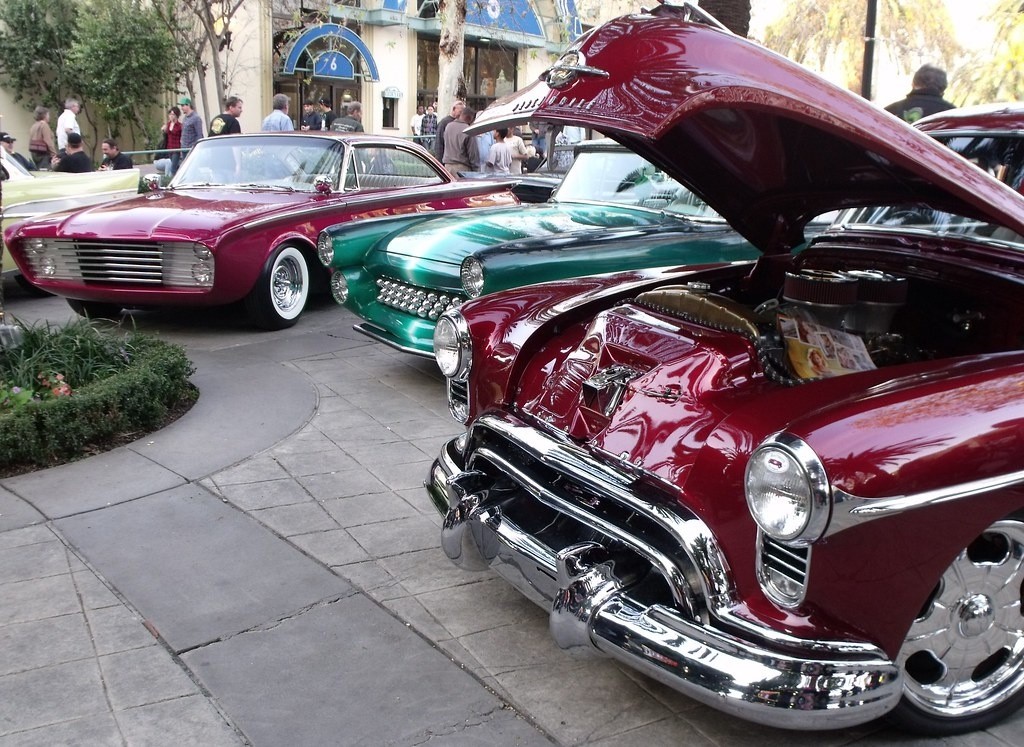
[29,138,48,152]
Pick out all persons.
[158,97,203,175]
[881,64,957,126]
[261,93,295,132]
[205,96,243,137]
[408,101,587,181]
[301,93,367,135]
[0,99,91,174]
[96,140,134,171]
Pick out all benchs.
[287,174,439,194]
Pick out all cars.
[4,132,522,331]
[0,137,140,299]
[247,135,845,360]
[422,0,1024,737]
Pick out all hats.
[0,133,16,141]
[319,97,331,107]
[176,98,191,106]
